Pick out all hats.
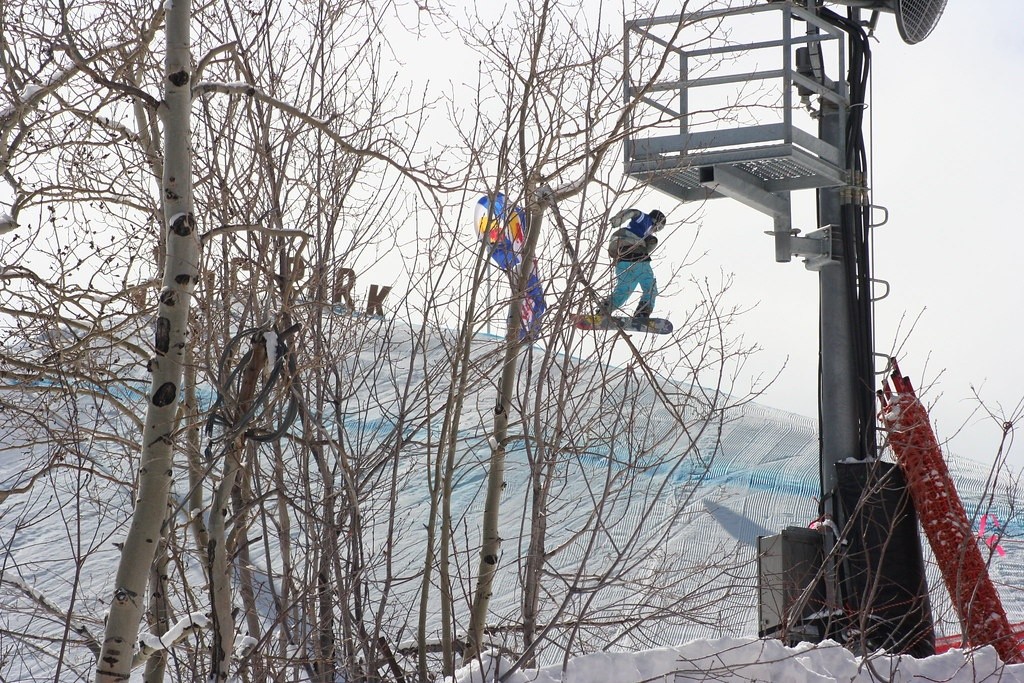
[648,210,666,224]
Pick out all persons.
[596,209,666,317]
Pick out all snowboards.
[568,312,673,335]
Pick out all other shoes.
[633,301,654,318]
[595,299,613,316]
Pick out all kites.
[474,165,545,342]
